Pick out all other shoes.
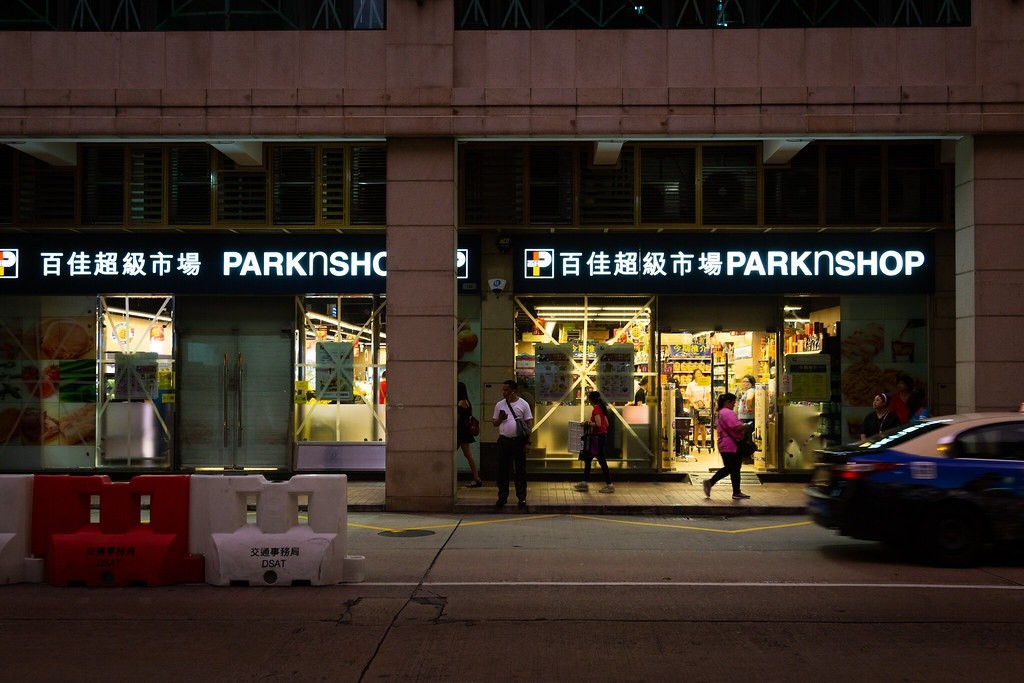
[496,498,507,505]
[466,479,482,487]
[518,498,526,505]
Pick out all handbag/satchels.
[516,420,529,440]
[469,416,479,436]
[579,422,593,461]
[735,440,757,458]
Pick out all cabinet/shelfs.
[713,363,735,381]
[668,358,713,401]
[572,357,598,400]
[758,359,770,377]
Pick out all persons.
[492,380,534,506]
[886,376,914,425]
[860,392,900,441]
[906,385,928,422]
[685,368,716,451]
[738,374,756,464]
[574,391,616,493]
[634,377,648,406]
[457,382,484,488]
[703,393,750,498]
[666,377,689,462]
[613,380,640,406]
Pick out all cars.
[802,412,1024,555]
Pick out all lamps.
[150,319,165,341]
[315,320,359,357]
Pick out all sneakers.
[732,492,750,499]
[703,480,712,497]
[599,486,614,493]
[574,484,588,492]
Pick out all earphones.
[512,392,515,395]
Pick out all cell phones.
[748,420,753,425]
[500,410,505,415]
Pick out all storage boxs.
[784,322,825,352]
